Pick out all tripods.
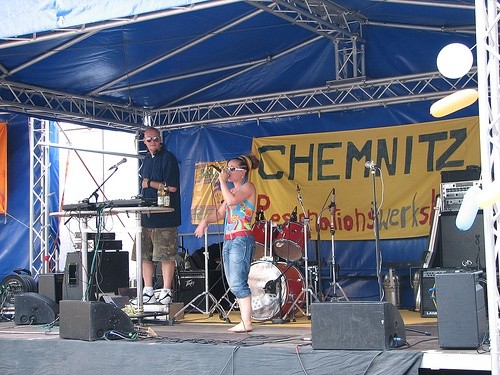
[171,227,232,324]
[282,190,349,320]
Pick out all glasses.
[146,136,161,142]
[226,167,247,173]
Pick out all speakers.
[174,270,239,312]
[310,301,406,351]
[13,250,134,340]
[419,211,490,350]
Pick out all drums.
[247,260,305,321]
[272,221,312,263]
[250,220,276,261]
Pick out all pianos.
[61,198,158,210]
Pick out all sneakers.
[156,293,172,305]
[129,293,157,304]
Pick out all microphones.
[109,158,127,170]
[364,160,379,169]
[290,206,297,222]
[330,188,336,215]
[257,206,264,222]
[267,285,273,290]
[211,165,222,173]
[296,184,302,201]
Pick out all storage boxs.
[179,270,228,313]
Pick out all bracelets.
[147,180,151,187]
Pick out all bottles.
[157,184,173,208]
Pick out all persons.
[194,155,259,333]
[130,128,180,304]
